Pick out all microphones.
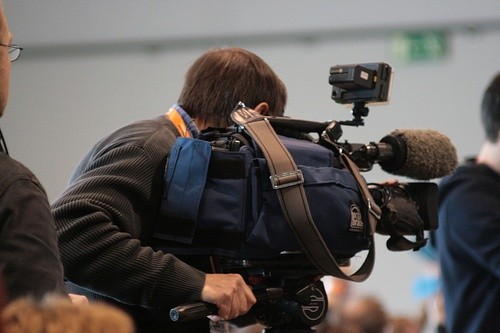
[335,129,459,182]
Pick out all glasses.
[0,44,23,63]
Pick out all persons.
[0,288,135,333]
[429,72,500,332]
[0,1,88,307]
[326,264,359,326]
[46,45,284,333]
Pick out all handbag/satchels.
[151,106,381,283]
